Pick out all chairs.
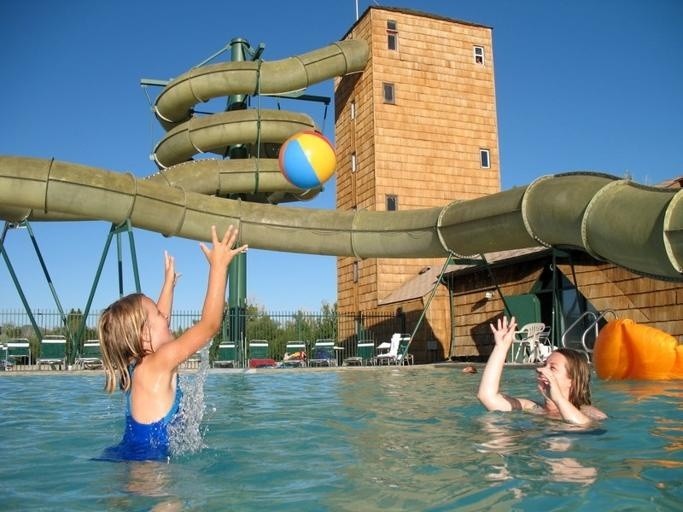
[185,333,415,367]
[0,333,105,370]
[512,322,545,363]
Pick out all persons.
[87,222,248,461]
[477,315,608,426]
[462,366,477,374]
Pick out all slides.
[0,38,683,284]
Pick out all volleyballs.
[280,131,337,188]
